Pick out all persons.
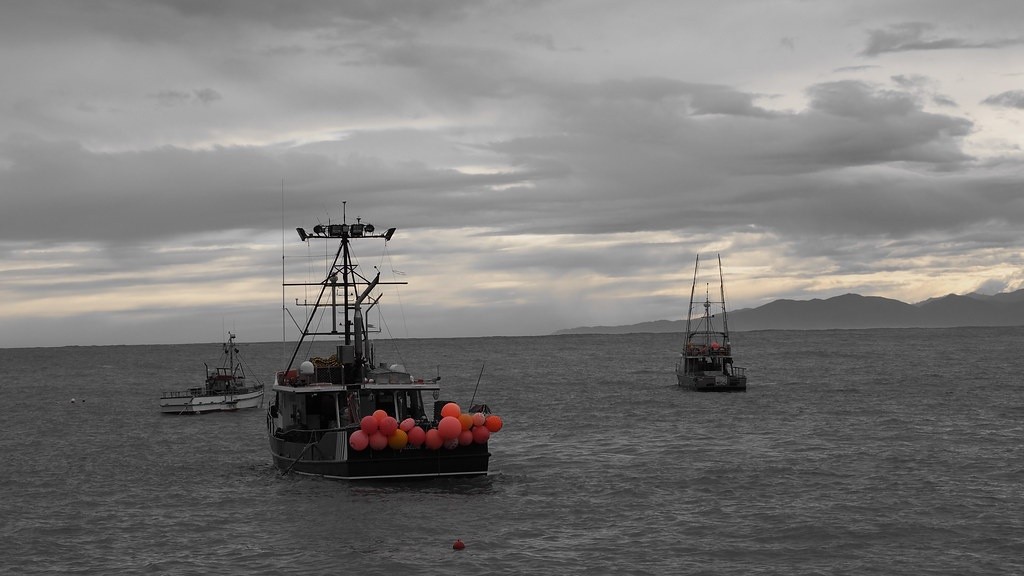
[209,376,215,396]
[697,344,707,354]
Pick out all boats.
[675,254,749,394]
[261,177,504,482]
[157,321,265,413]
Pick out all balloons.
[350,402,503,451]
